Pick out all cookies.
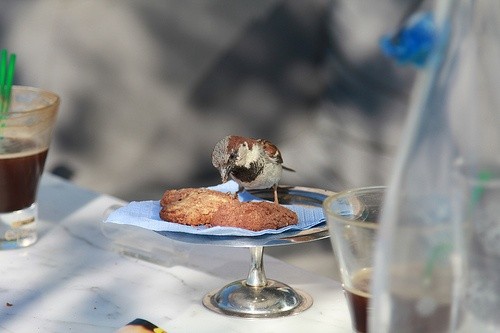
[158,187,236,225]
[209,201,297,231]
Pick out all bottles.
[365,1,500,333]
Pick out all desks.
[0,172,356,333]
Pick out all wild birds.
[211,136,295,208]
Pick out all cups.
[0,85,60,250]
[321,187,456,333]
